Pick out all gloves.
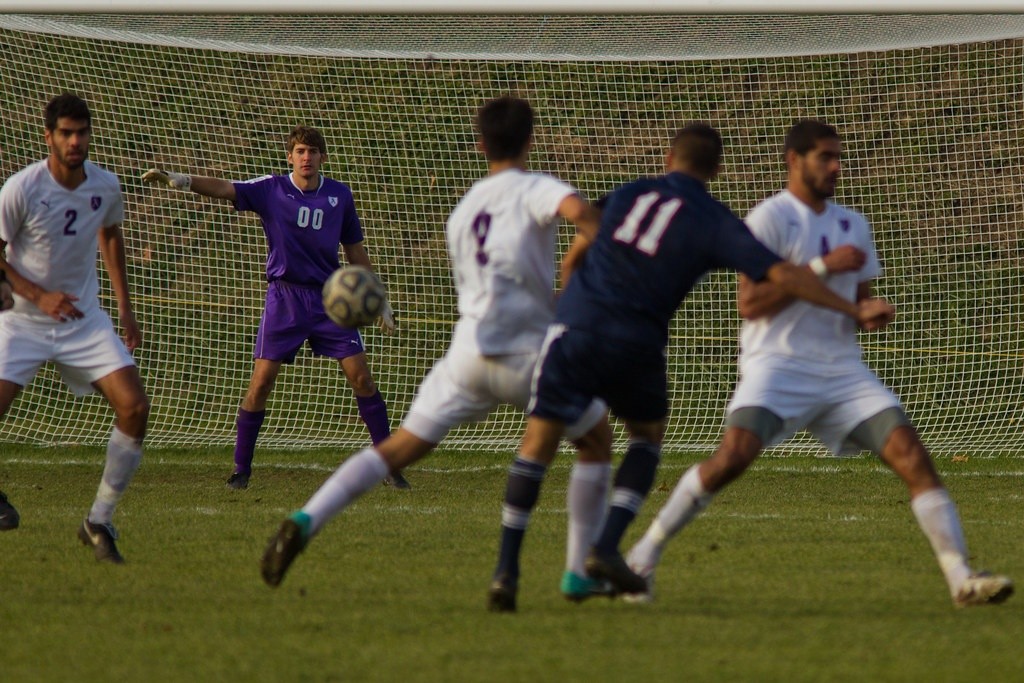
[141,168,192,193]
[377,298,395,336]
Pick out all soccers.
[322,264,387,329]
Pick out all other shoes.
[559,571,601,599]
[383,471,413,489]
[953,575,1014,610]
[487,580,515,613]
[586,555,646,593]
[227,470,248,491]
[261,512,310,588]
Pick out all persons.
[485,122,897,609]
[261,95,612,599]
[0,92,142,567]
[140,124,414,491]
[621,118,1016,613]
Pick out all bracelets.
[808,256,827,276]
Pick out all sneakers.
[0,492,19,531]
[77,518,123,566]
